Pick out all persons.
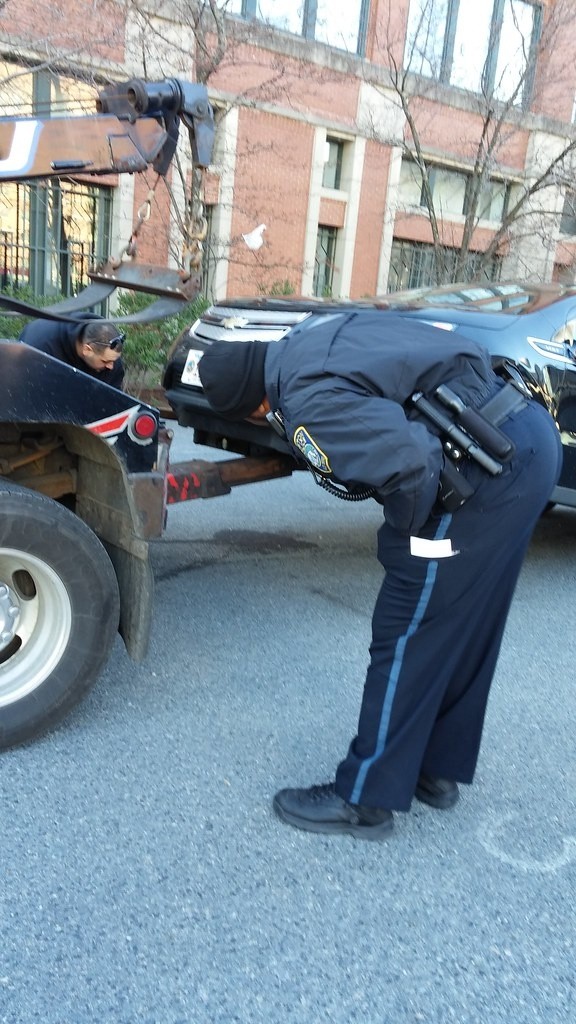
[198,312,563,840]
[19,311,125,393]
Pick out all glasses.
[87,333,126,349]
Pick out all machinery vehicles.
[0,76,291,753]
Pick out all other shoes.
[414,769,459,809]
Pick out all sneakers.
[273,782,394,840]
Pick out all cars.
[161,279,576,509]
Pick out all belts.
[479,383,524,426]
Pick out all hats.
[198,340,267,421]
